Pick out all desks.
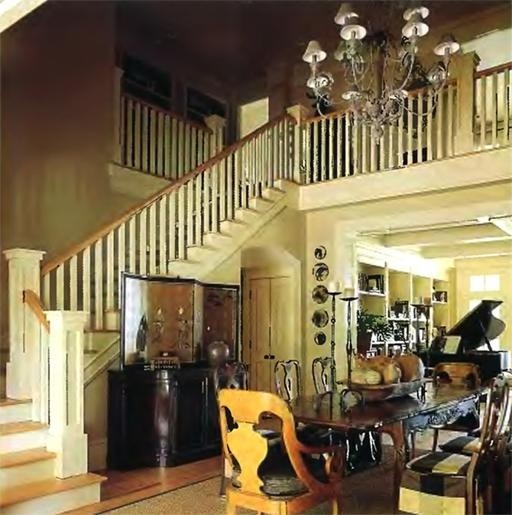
[282,385,480,513]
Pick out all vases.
[207,341,230,367]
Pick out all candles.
[328,270,356,298]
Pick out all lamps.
[303,1,460,144]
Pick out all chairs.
[397,372,511,514]
[312,356,383,465]
[424,362,483,452]
[440,372,509,514]
[275,359,353,479]
[219,389,346,515]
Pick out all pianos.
[414,300,508,385]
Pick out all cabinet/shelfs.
[110,365,249,470]
[357,244,461,359]
